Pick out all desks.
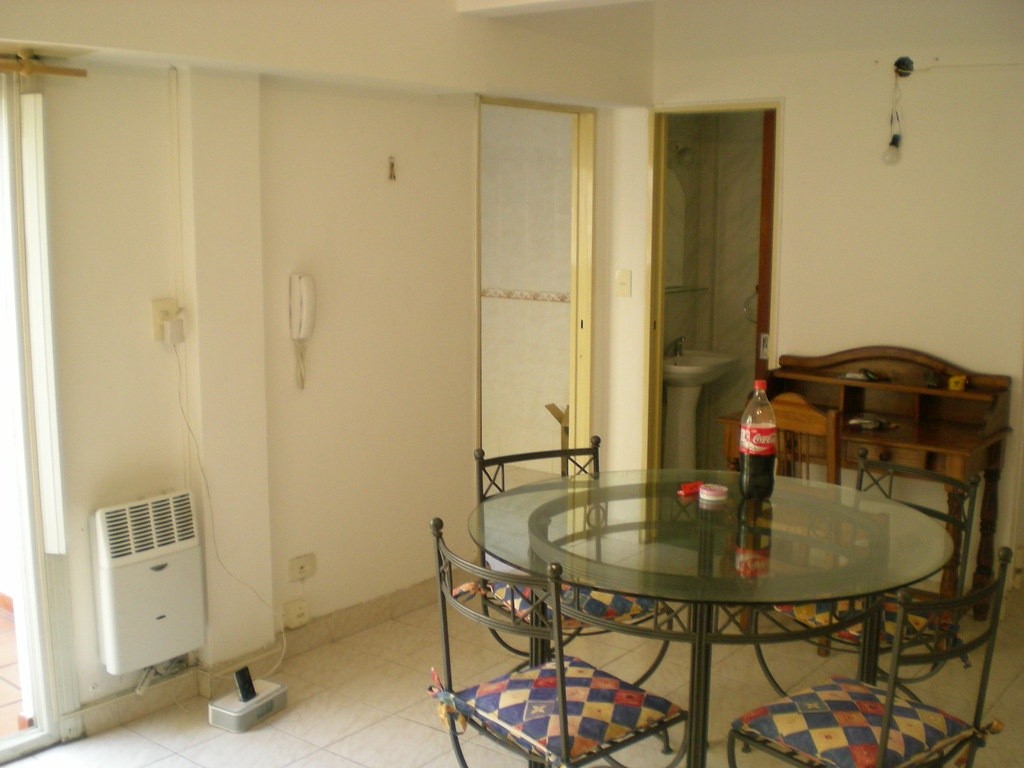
[715,345,1013,659]
[466,469,953,768]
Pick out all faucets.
[674,336,688,356]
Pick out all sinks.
[663,347,741,386]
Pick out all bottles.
[737,380,777,500]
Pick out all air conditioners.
[91,489,202,675]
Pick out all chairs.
[430,518,690,768]
[741,395,890,657]
[742,449,982,701]
[727,546,1011,768]
[472,437,677,685]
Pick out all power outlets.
[152,296,177,344]
